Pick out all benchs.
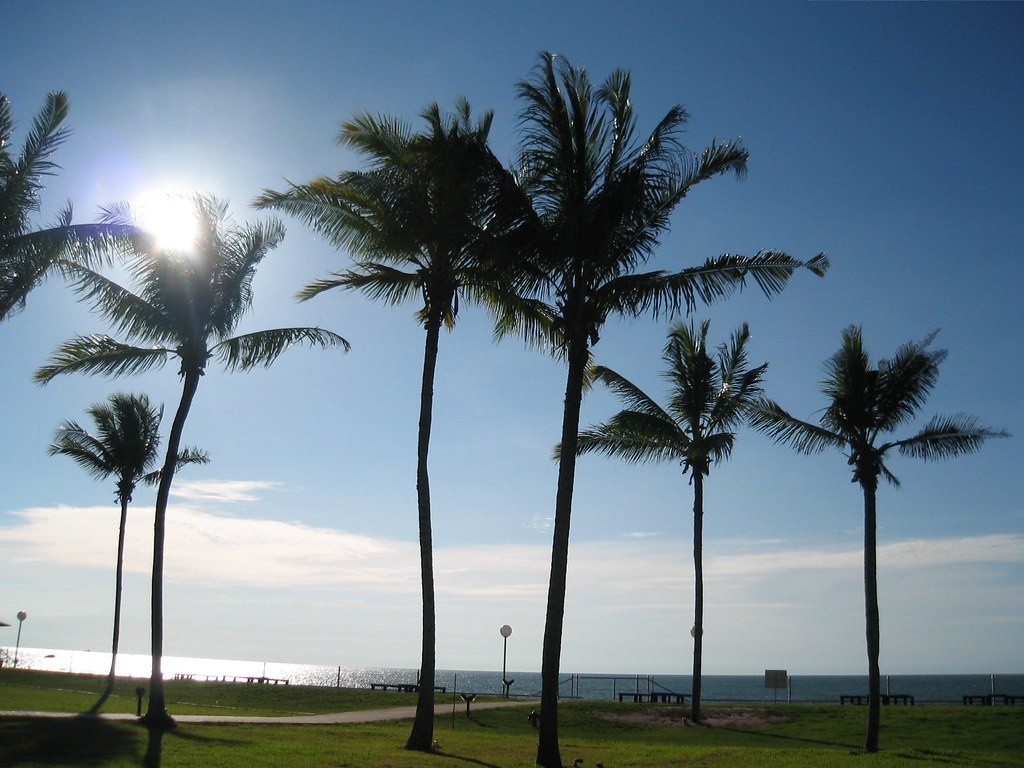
[962,694,1024,706]
[223,675,238,682]
[840,694,914,706]
[175,674,196,680]
[371,683,445,694]
[651,692,685,703]
[239,677,289,686]
[206,676,218,681]
[618,693,650,702]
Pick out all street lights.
[499,624,512,695]
[13,611,27,668]
